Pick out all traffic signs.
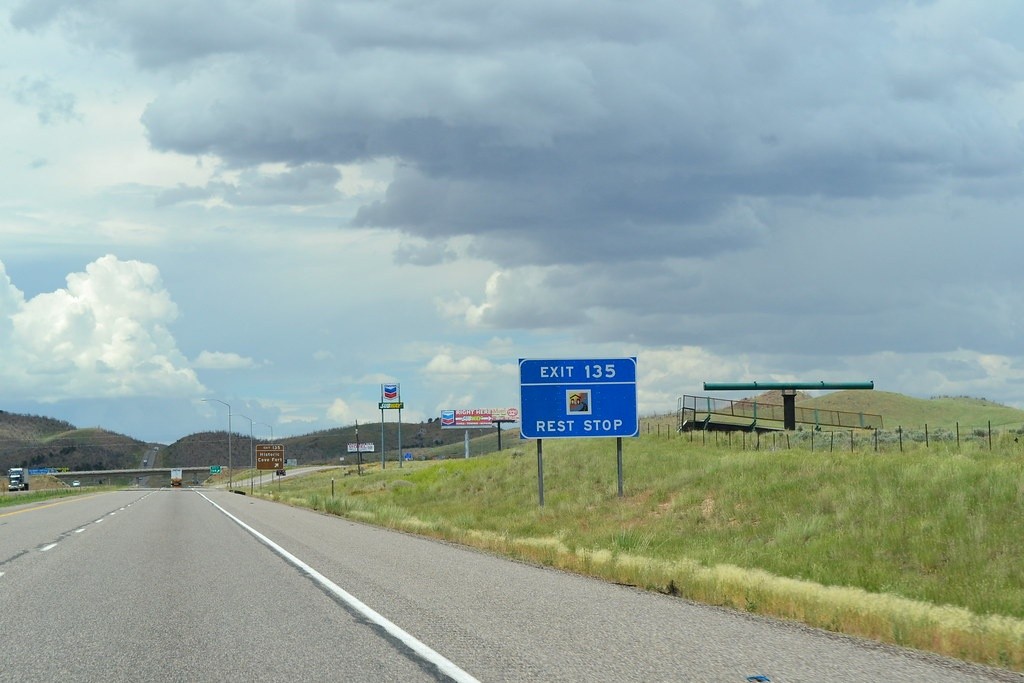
[256,444,284,471]
[210,465,221,473]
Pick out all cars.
[72,481,80,487]
[276,469,286,476]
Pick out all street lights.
[229,414,253,495]
[252,422,274,482]
[201,398,232,488]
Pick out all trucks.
[8,468,29,491]
[170,469,183,487]
[28,469,58,475]
[46,467,70,473]
